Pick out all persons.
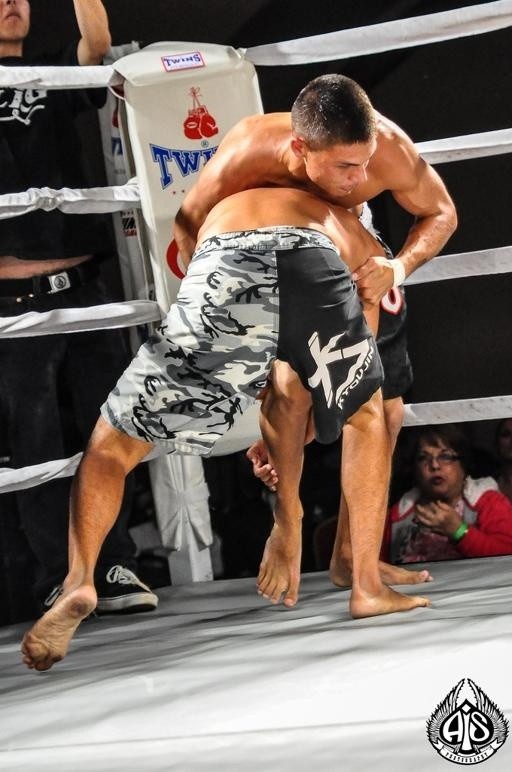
[170,67,464,593]
[18,185,430,678]
[128,487,161,559]
[377,424,511,565]
[492,418,512,501]
[1,1,164,620]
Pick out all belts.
[0,256,104,301]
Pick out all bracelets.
[450,520,468,544]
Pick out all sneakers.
[95,564,160,623]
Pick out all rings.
[430,515,435,520]
